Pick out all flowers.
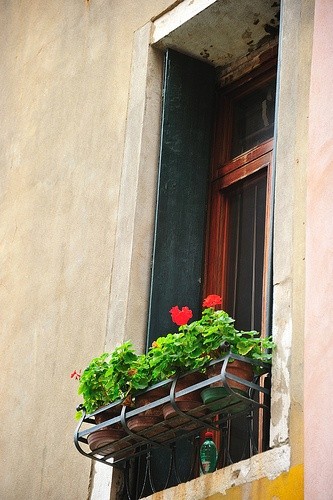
[70,353,123,410]
[112,345,156,389]
[201,293,256,358]
[152,303,210,370]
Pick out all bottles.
[199,432,217,477]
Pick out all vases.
[125,393,169,432]
[202,358,256,404]
[168,372,210,412]
[88,401,126,454]
